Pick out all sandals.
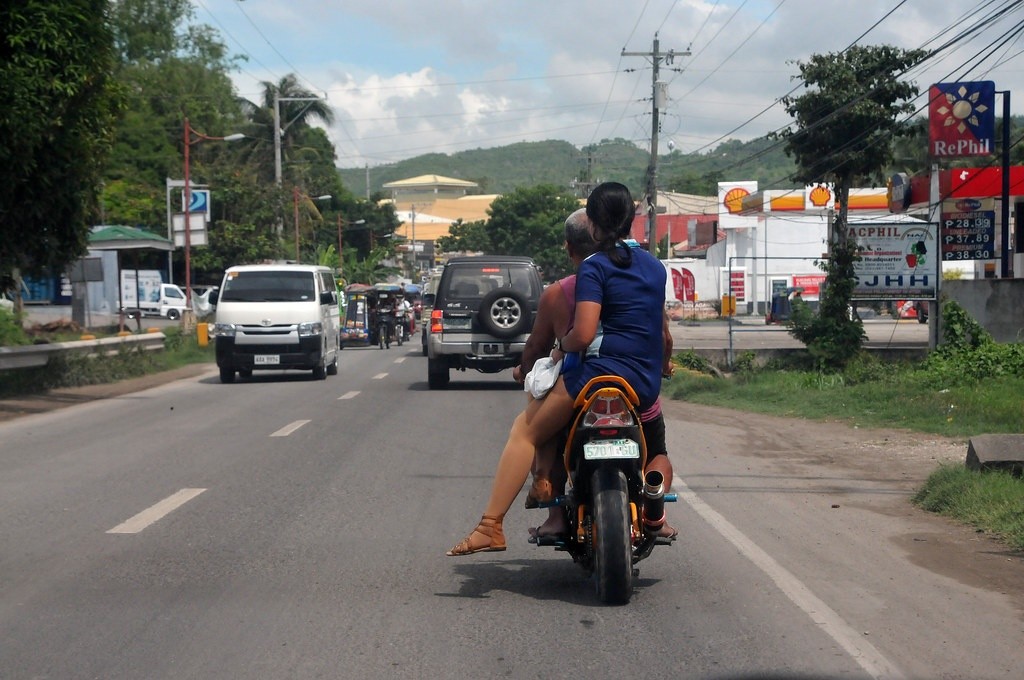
[523,468,557,510]
[444,512,508,555]
[525,524,565,544]
[654,526,680,540]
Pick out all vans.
[215,266,340,382]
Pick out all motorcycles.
[341,283,421,349]
[535,377,679,606]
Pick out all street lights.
[369,230,392,252]
[336,213,365,266]
[292,185,332,265]
[178,116,245,334]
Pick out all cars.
[183,284,220,318]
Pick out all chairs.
[504,284,528,294]
[455,284,479,296]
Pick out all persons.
[512,208,679,543]
[375,295,421,343]
[489,278,499,290]
[509,276,529,293]
[445,182,668,556]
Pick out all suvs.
[422,256,549,392]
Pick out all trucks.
[116,283,186,321]
